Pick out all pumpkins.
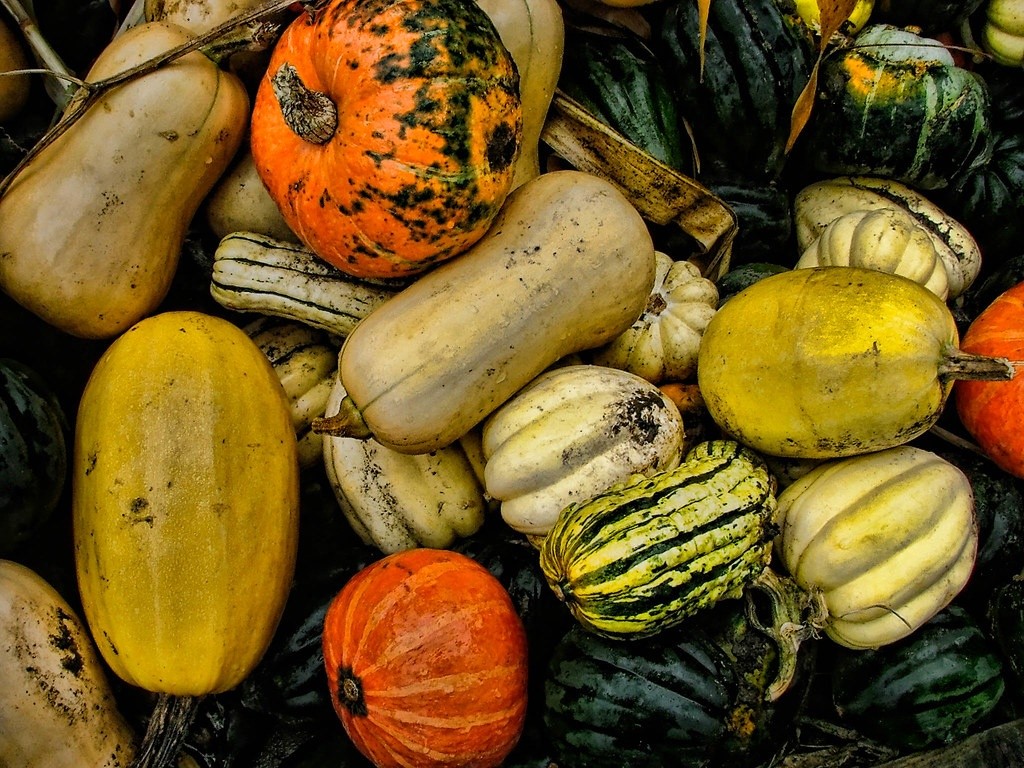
[0,0,1024,768]
